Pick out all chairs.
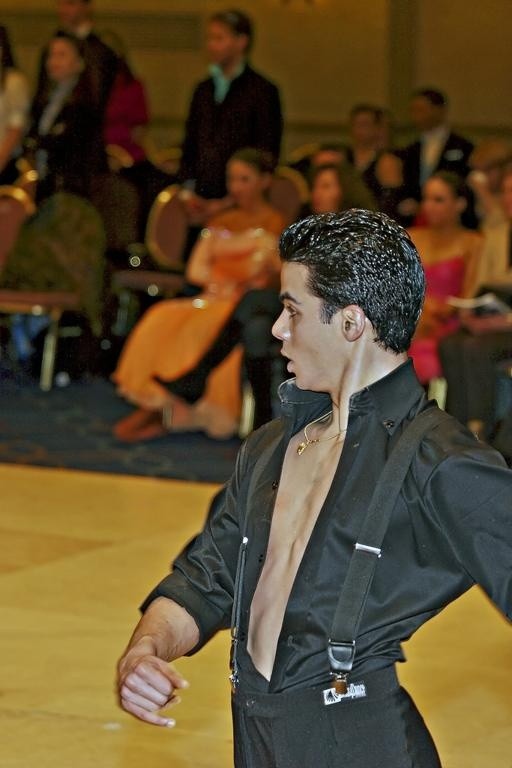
[0,145,318,393]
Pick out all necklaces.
[297,412,347,456]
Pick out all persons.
[114,88,512,461]
[119,207,512,768]
[176,10,285,201]
[0,0,151,200]
